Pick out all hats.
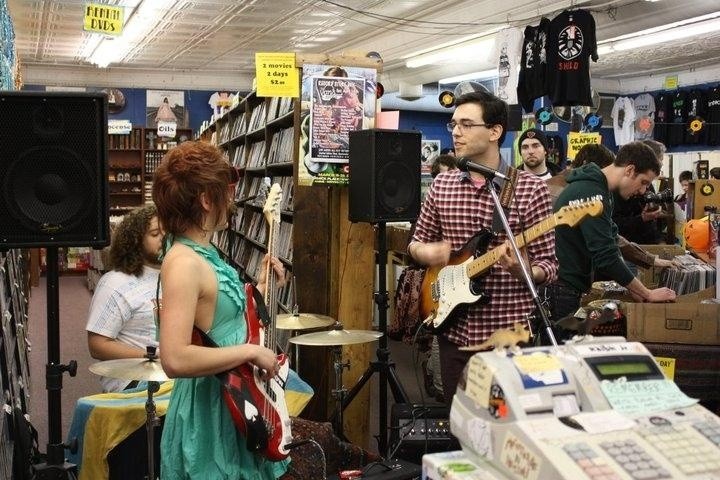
[517,129,548,150]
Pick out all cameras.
[630,188,673,213]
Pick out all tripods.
[30,249,79,480]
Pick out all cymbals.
[274,303,337,330]
[86,344,173,382]
[286,321,386,347]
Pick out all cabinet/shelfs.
[193,53,379,455]
[38,127,197,274]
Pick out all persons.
[515,127,563,180]
[672,170,692,210]
[612,140,669,246]
[537,141,663,345]
[155,97,177,122]
[152,140,291,480]
[86,205,166,394]
[407,93,557,398]
[706,165,720,179]
[545,143,616,212]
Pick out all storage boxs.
[625,245,720,343]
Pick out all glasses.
[446,122,497,132]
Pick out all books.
[108,128,141,149]
[277,222,294,260]
[218,122,229,142]
[144,153,169,173]
[275,267,294,311]
[246,248,266,278]
[271,176,293,212]
[267,127,295,164]
[659,251,717,296]
[245,141,267,168]
[246,211,268,244]
[230,208,245,231]
[231,144,245,168]
[267,97,294,122]
[211,230,230,255]
[274,307,292,353]
[231,112,246,138]
[208,132,216,145]
[247,102,266,132]
[234,175,271,206]
[228,236,248,268]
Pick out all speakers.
[349,129,421,225]
[326,222,412,459]
[0,91,111,251]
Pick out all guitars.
[417,194,605,335]
[222,182,293,461]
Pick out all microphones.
[457,156,511,183]
